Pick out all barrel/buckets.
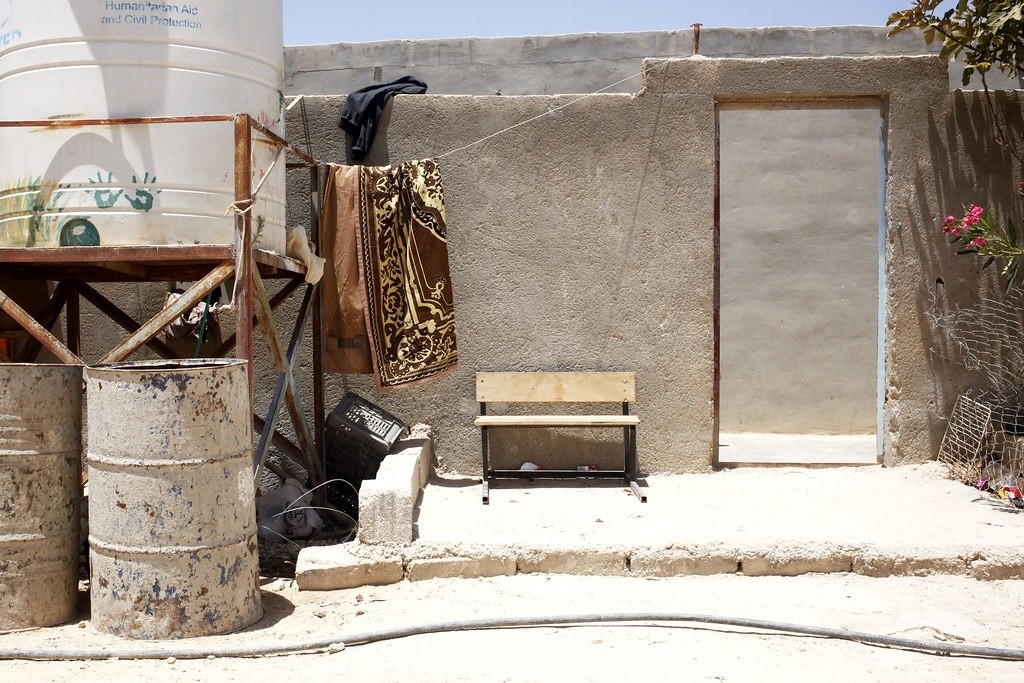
[83,356,264,636]
[0,363,85,630]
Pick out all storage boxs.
[323,389,406,508]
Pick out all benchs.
[474,371,647,505]
